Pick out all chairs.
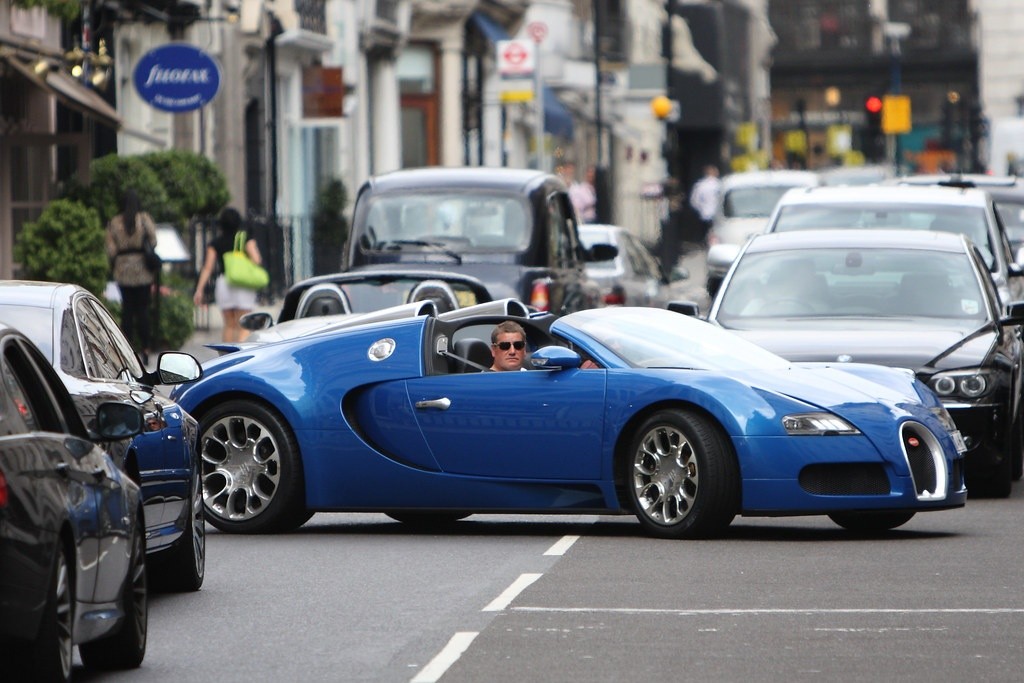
[455,337,495,372]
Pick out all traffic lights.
[865,96,882,115]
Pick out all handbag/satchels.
[223,231,269,288]
[145,253,161,269]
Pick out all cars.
[0,276,209,683]
[707,164,1024,317]
[237,164,689,325]
[706,232,1024,499]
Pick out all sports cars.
[169,296,969,541]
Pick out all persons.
[193,207,261,343]
[481,321,598,372]
[103,191,157,364]
[561,162,596,224]
[688,165,721,222]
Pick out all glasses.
[493,341,525,351]
